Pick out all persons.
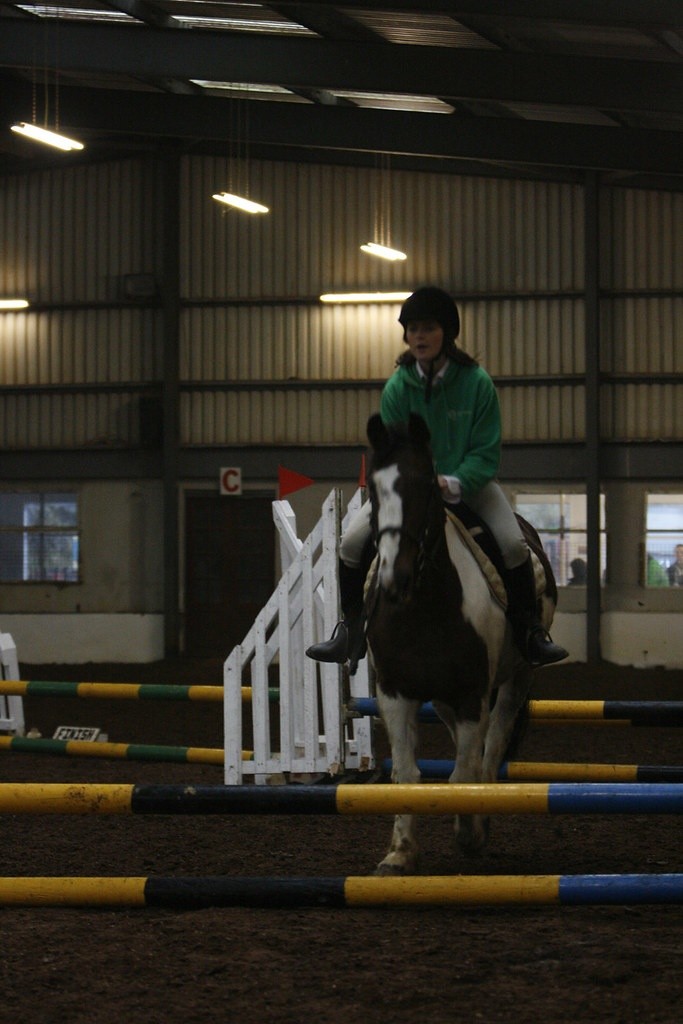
[565,558,589,587]
[304,283,571,666]
[646,545,683,587]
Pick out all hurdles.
[0,669,682,910]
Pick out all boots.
[505,549,569,666]
[305,556,367,663]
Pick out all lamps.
[358,154,408,263]
[6,0,86,154]
[212,29,270,216]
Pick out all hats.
[398,286,460,339]
